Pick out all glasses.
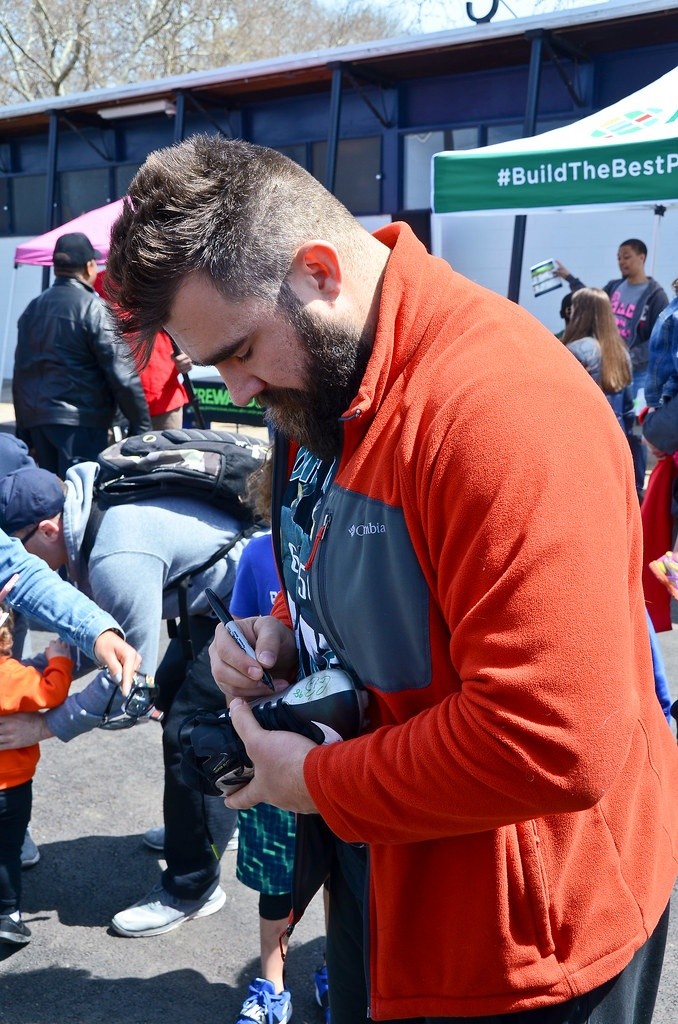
[96,671,160,730]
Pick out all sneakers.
[0,915,32,943]
[180,667,372,799]
[315,966,331,1024]
[112,881,226,938]
[20,829,41,867]
[144,826,166,849]
[238,978,293,1024]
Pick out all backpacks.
[80,427,271,575]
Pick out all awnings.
[0,194,131,396]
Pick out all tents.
[431,65,678,257]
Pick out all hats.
[53,233,101,266]
[0,467,64,534]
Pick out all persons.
[0,526,142,698]
[0,608,75,944]
[92,270,192,430]
[12,232,153,482]
[640,279,678,603]
[229,455,331,1024]
[0,434,36,480]
[561,287,633,437]
[101,131,678,1024]
[0,429,272,939]
[551,238,669,508]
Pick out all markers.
[203,587,277,692]
